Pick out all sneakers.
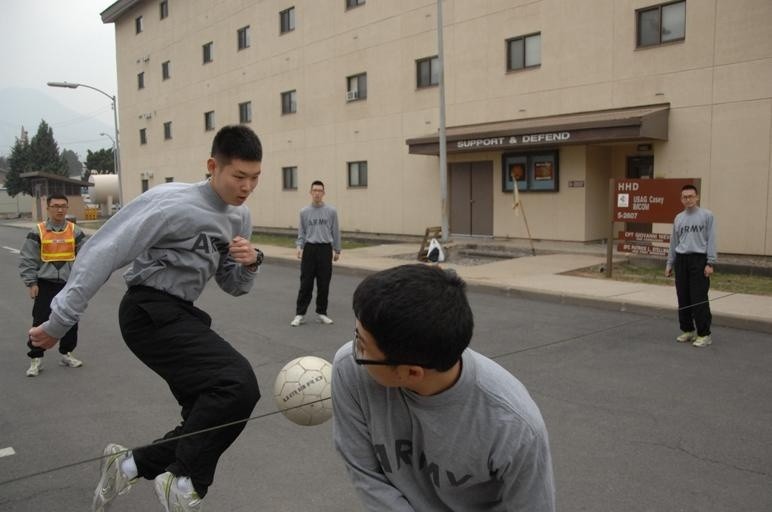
[290,312,334,326]
[26,351,83,377]
[92,443,202,512]
[676,330,713,348]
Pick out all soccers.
[274,356,332,426]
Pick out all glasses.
[680,195,697,201]
[350,334,434,370]
[48,205,69,211]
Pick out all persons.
[30,126,262,511]
[19,193,90,376]
[330,264,557,512]
[290,180,340,328]
[665,184,717,347]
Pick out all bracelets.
[250,248,264,265]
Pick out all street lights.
[97,129,116,175]
[46,81,123,209]
